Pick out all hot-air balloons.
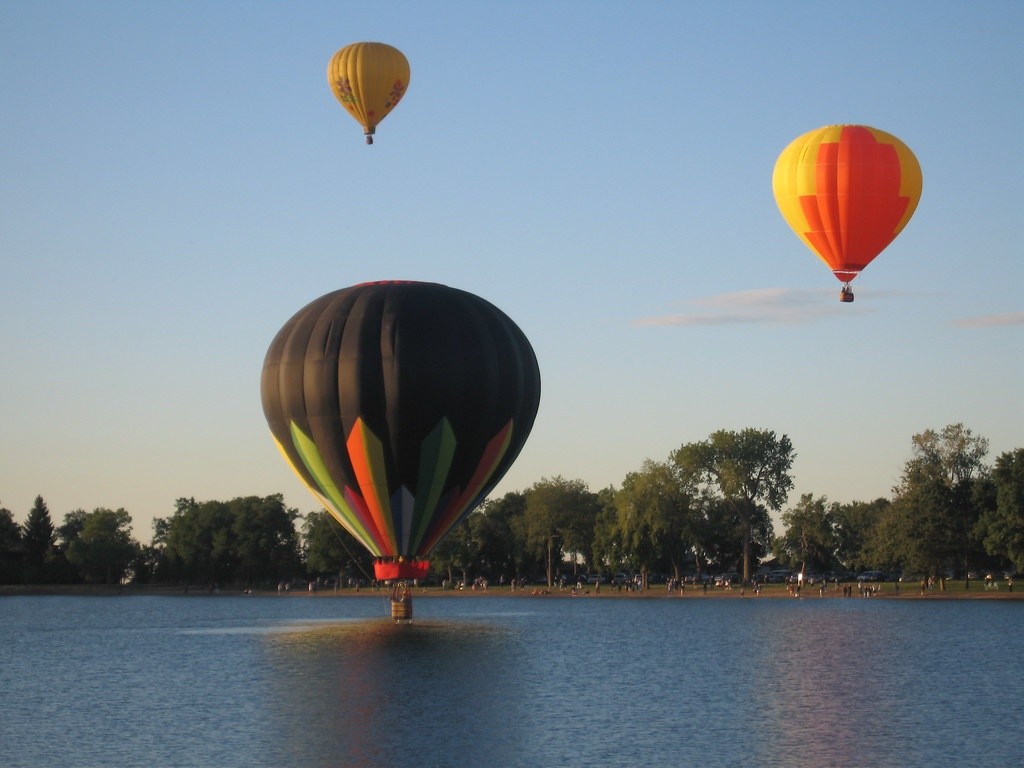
[769,121,924,311]
[329,41,410,143]
[258,278,543,625]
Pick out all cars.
[968,571,982,580]
[857,571,882,583]
[587,573,660,585]
[689,573,742,586]
[752,570,846,583]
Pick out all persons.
[1008,575,1015,592]
[611,575,643,592]
[208,579,220,593]
[442,576,505,593]
[751,573,769,595]
[553,577,602,595]
[509,576,552,596]
[855,581,881,598]
[919,574,934,596]
[665,574,732,592]
[842,286,850,294]
[785,575,852,598]
[305,576,428,594]
[277,581,291,592]
